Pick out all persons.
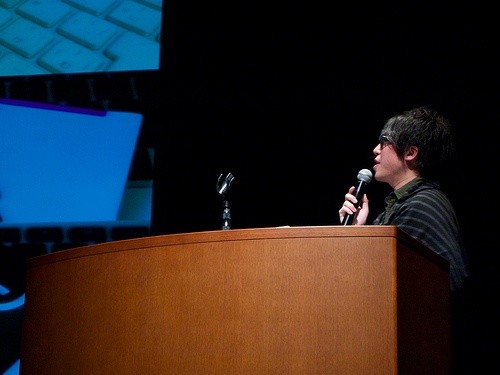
[339,106,473,375]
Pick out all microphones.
[342,169,373,226]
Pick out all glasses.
[380,135,392,146]
